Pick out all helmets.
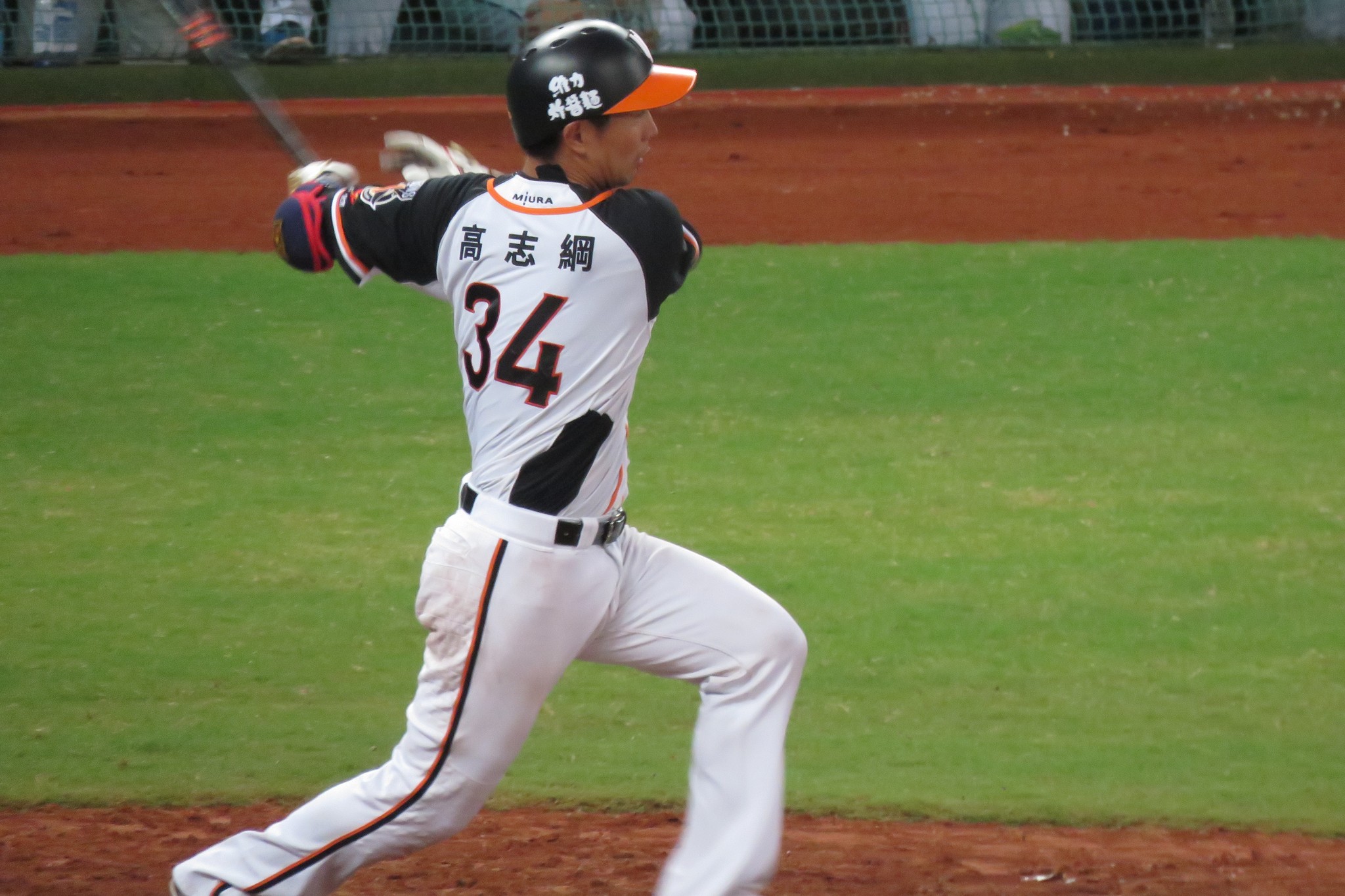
[506,18,696,145]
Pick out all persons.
[12,0,1071,68]
[168,19,808,896]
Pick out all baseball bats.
[158,0,357,192]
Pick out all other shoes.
[264,20,314,58]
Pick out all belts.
[460,484,627,544]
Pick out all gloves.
[287,159,360,192]
[381,132,486,181]
